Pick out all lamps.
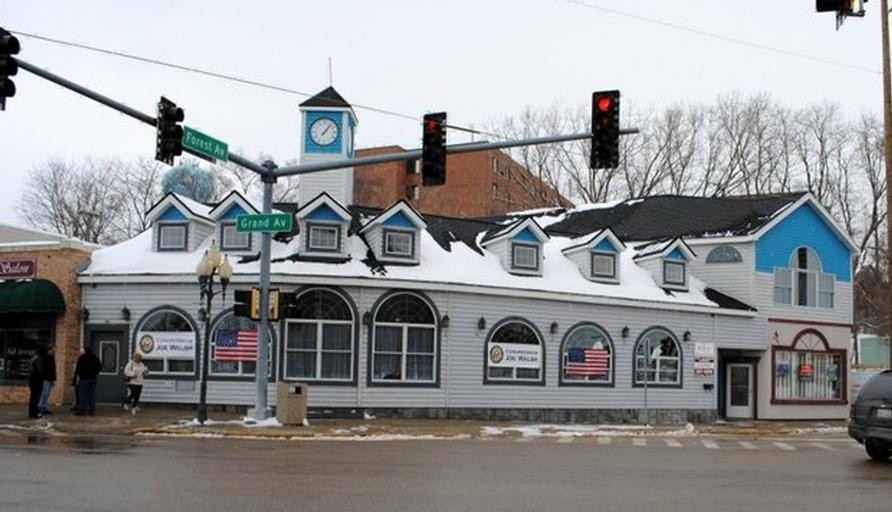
[683,329,691,342]
[551,321,559,334]
[622,325,630,338]
[478,316,487,330]
[362,310,453,328]
[79,305,130,322]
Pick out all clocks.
[308,115,340,148]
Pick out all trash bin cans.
[275,383,308,425]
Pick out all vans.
[844,366,892,461]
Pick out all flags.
[565,347,608,376]
[214,327,259,361]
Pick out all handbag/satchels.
[119,370,129,380]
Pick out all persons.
[29,341,47,418]
[72,343,102,417]
[70,345,87,411]
[122,353,151,415]
[37,343,58,415]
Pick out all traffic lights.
[588,88,622,168]
[421,109,449,189]
[0,25,22,113]
[269,287,300,322]
[153,95,188,166]
[230,286,257,319]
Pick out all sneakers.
[31,411,51,418]
[70,407,93,416]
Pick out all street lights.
[192,236,236,423]
[813,0,892,370]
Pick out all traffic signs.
[235,212,294,233]
[180,124,229,163]
